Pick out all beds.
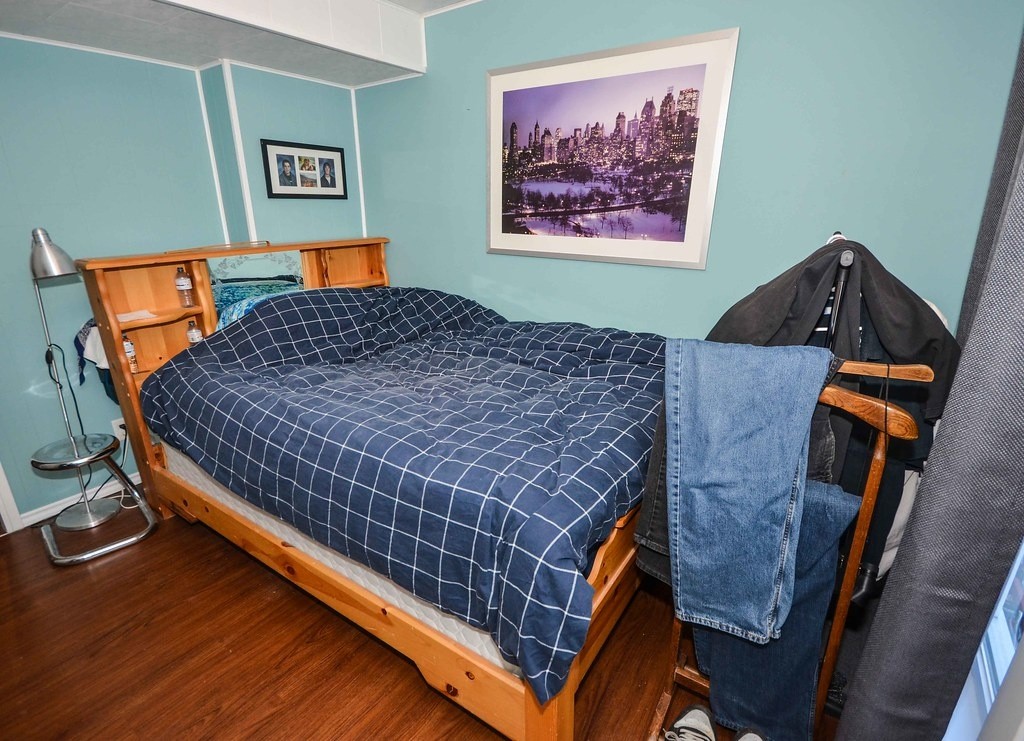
[74,237,665,741]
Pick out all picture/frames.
[260,138,348,199]
[485,26,741,270]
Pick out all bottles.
[122,333,139,374]
[186,321,203,345]
[175,268,194,307]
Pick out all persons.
[279,160,297,186]
[319,162,336,187]
[299,159,314,171]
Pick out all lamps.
[28,227,157,565]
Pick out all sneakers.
[732,728,768,741]
[661,704,717,741]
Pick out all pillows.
[212,281,302,319]
[216,286,361,338]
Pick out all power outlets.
[111,418,130,441]
